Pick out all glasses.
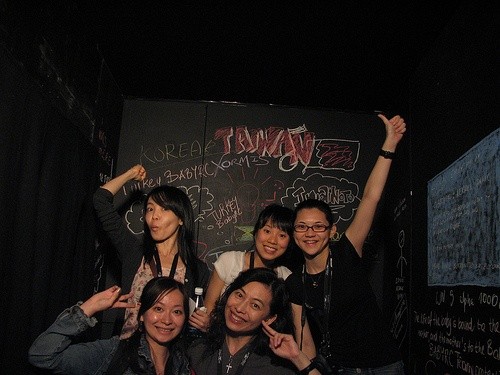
[293,223,330,232]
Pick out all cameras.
[316,337,344,375]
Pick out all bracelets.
[379,149,395,159]
[300,363,315,375]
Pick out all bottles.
[188,287,207,338]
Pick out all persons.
[284,114,408,375]
[193,267,325,374]
[93,165,210,374]
[205,204,296,319]
[28,276,195,375]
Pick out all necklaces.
[154,246,179,279]
[302,248,333,358]
[250,249,277,270]
